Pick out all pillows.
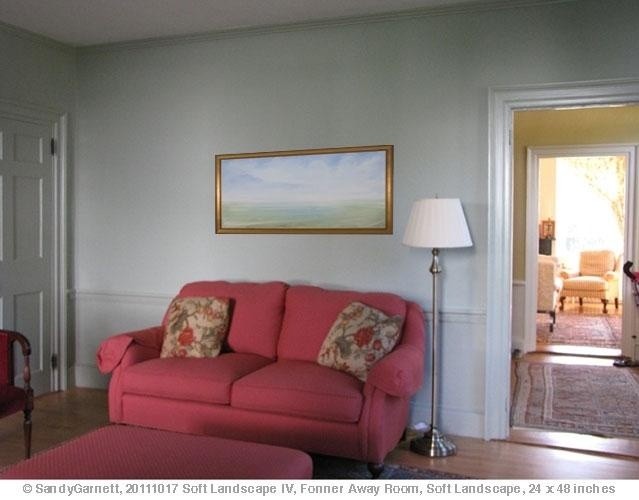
[316,301,403,383]
[160,297,234,358]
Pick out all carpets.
[536,312,623,347]
[307,449,479,479]
[508,359,639,442]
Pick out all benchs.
[1,422,314,479]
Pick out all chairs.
[559,251,623,314]
[0,328,35,460]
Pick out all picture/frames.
[542,220,555,239]
[215,145,393,233]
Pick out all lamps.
[401,195,474,456]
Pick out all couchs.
[94,280,425,479]
[537,255,559,331]
